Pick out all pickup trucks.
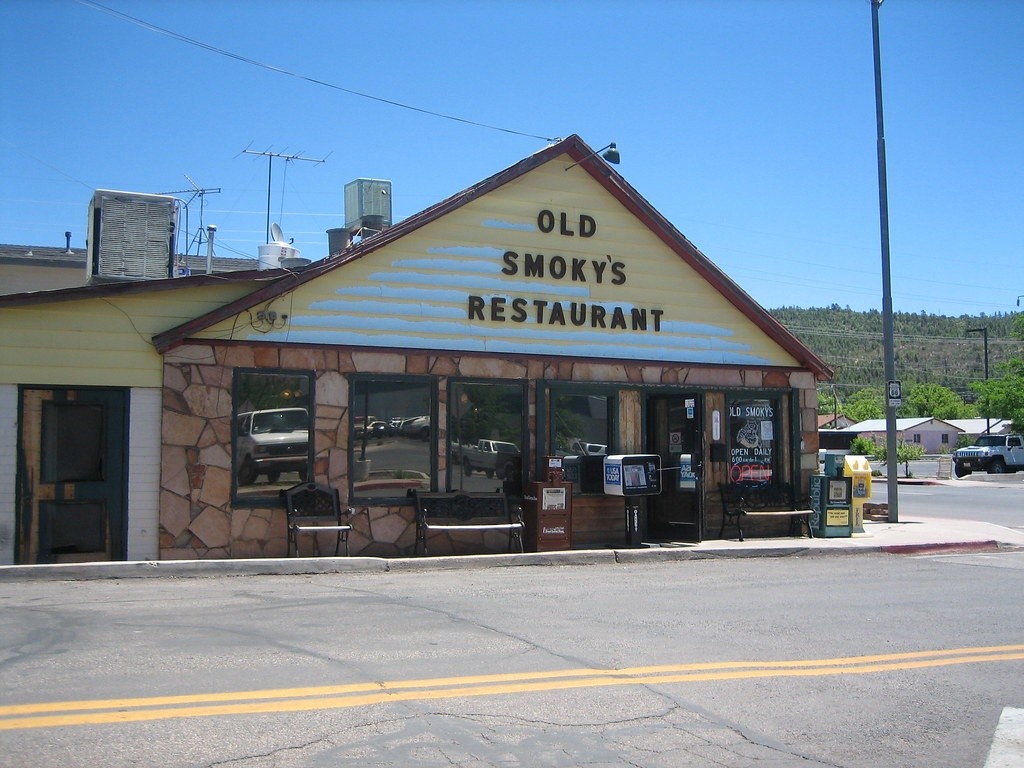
[453,440,522,480]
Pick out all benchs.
[714,478,816,543]
[411,489,527,556]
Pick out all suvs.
[571,441,608,455]
[951,432,1024,477]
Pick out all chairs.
[283,481,354,559]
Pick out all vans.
[237,408,311,486]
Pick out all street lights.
[967,328,990,436]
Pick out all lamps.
[564,142,621,171]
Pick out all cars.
[741,461,772,482]
[356,415,432,443]
